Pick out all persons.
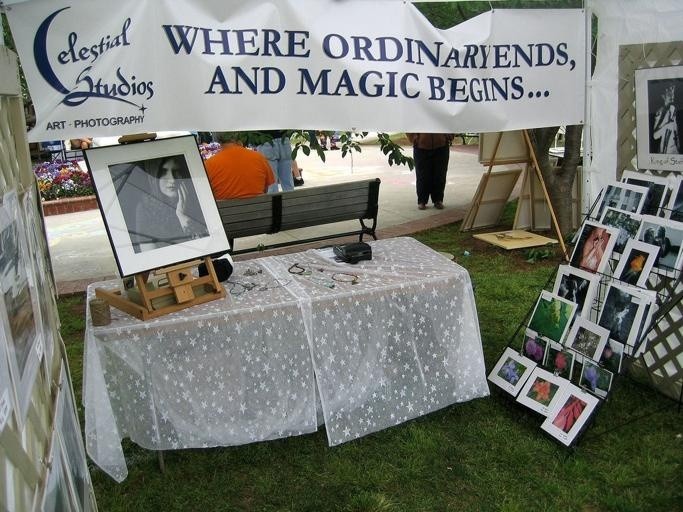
[135,156,209,253]
[204,131,275,199]
[405,133,455,209]
[653,86,680,154]
[247,131,294,193]
[291,159,304,186]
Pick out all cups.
[88,297,111,327]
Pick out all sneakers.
[294,177,305,187]
[329,146,340,151]
[416,203,426,210]
[433,200,444,209]
[321,147,328,152]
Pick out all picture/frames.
[81,132,234,280]
[634,64,683,171]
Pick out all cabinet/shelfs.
[477,183,682,457]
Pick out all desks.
[77,232,492,488]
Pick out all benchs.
[208,176,381,257]
